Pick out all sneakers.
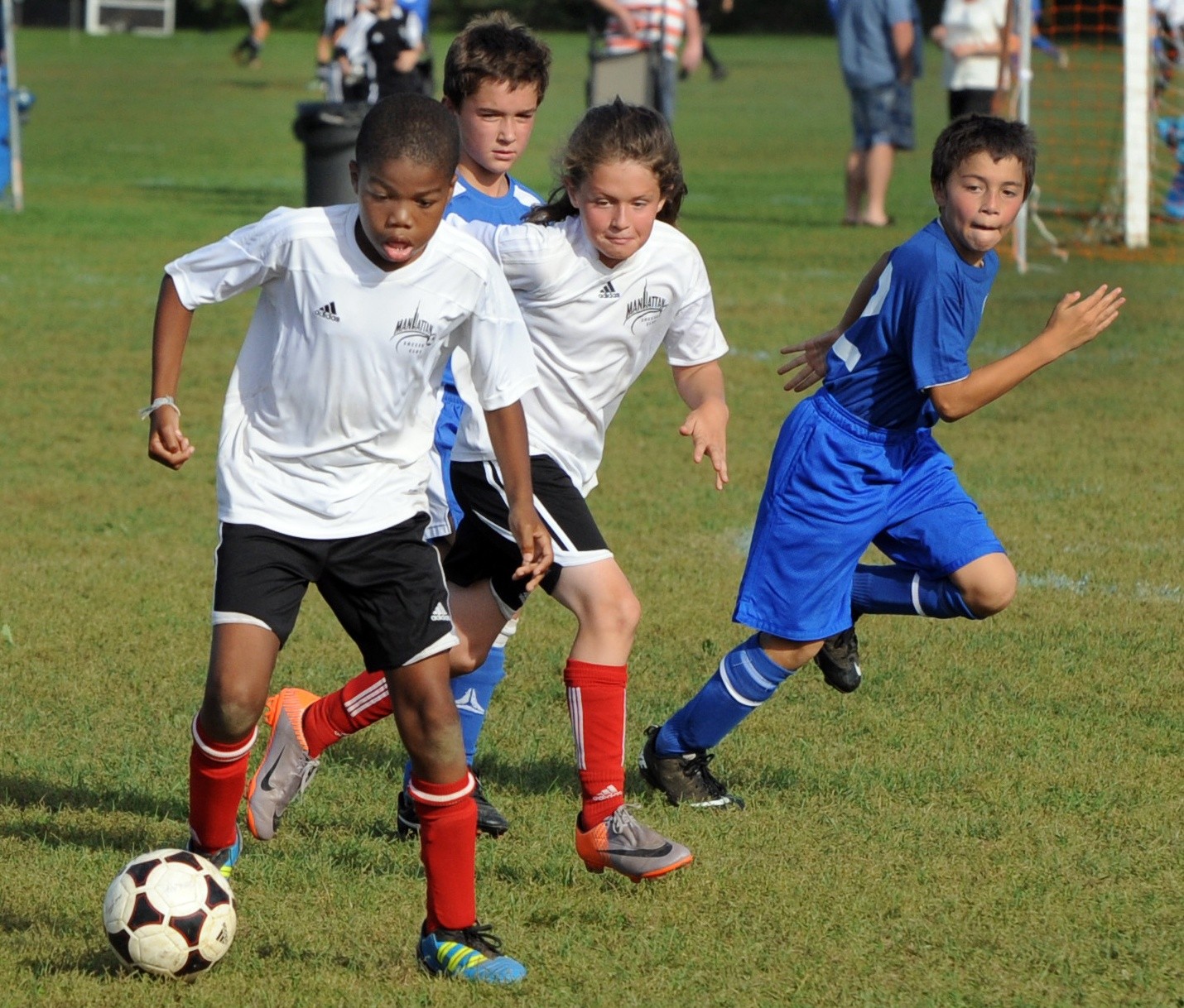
[574,804,692,883]
[416,917,527,983]
[244,687,322,841]
[814,623,862,692]
[398,774,508,837]
[639,724,745,810]
[186,822,243,881]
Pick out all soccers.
[102,847,237,981]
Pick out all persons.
[146,94,555,987]
[232,1,1184,840]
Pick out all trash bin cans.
[294,100,373,206]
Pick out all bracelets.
[139,398,180,419]
